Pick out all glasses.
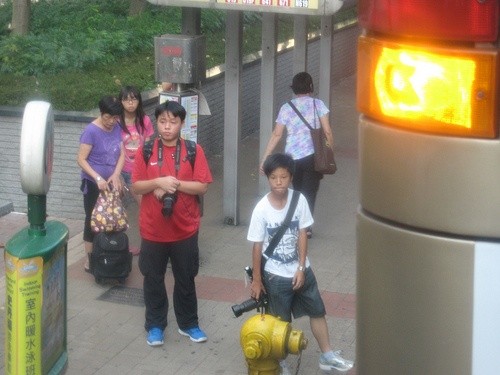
[123,97,140,104]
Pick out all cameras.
[160,193,176,216]
[231,291,268,318]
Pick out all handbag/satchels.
[91,189,130,234]
[89,235,131,282]
[312,126,338,174]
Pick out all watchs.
[297,266,306,272]
[95,176,103,181]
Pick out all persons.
[259,72,334,238]
[131,100,212,346]
[246,151,355,375]
[115,84,156,256]
[75,95,127,272]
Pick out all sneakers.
[147,328,166,346]
[317,350,353,372]
[177,326,207,343]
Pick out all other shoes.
[84,258,92,273]
[306,226,313,240]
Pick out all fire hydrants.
[238,313,309,375]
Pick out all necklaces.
[171,154,176,159]
